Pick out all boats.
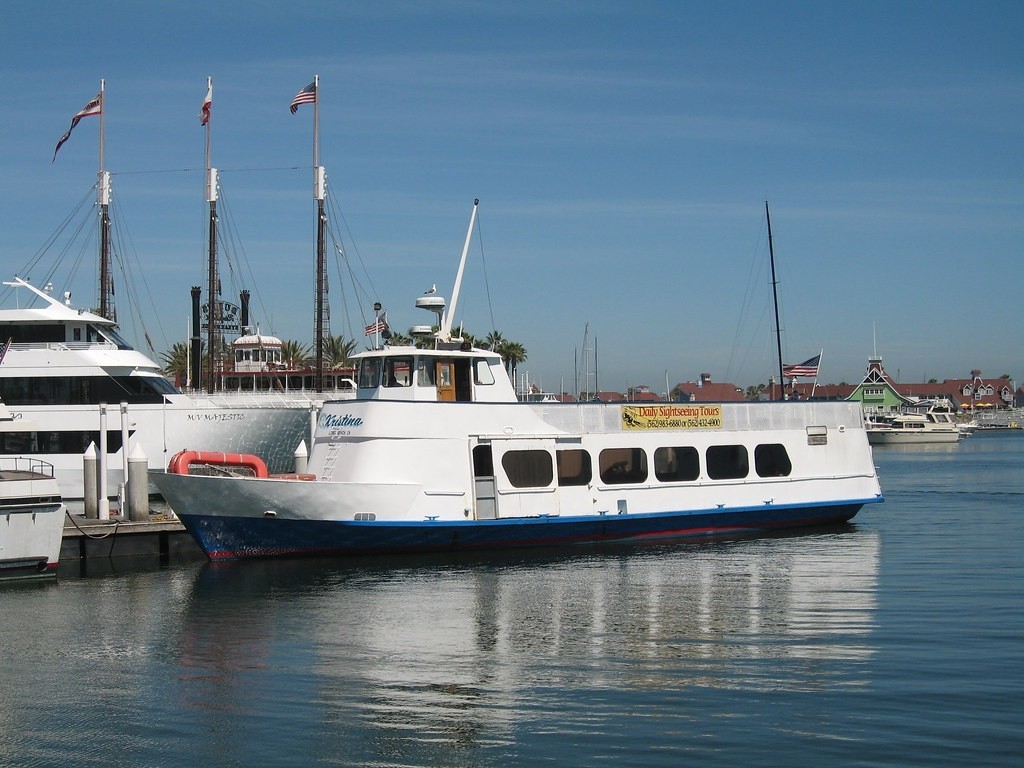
[866,414,964,445]
[955,419,980,438]
[145,196,883,572]
[0,457,65,588]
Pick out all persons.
[418,360,427,387]
[388,376,403,386]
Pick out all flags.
[782,354,821,379]
[51,79,104,165]
[197,77,213,127]
[289,75,318,117]
[0,337,12,366]
[364,309,387,336]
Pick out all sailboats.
[0,165,377,518]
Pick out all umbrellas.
[960,402,995,412]
